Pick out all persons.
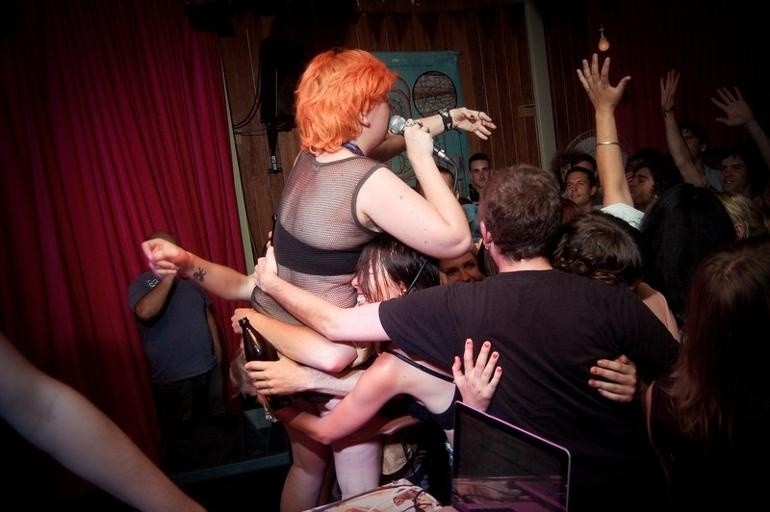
[0,336,207,512]
[127,51,770,510]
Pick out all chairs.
[452,400,573,512]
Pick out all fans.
[560,129,632,203]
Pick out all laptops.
[429,401,571,512]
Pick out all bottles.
[240,318,299,410]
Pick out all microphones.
[387,115,455,168]
